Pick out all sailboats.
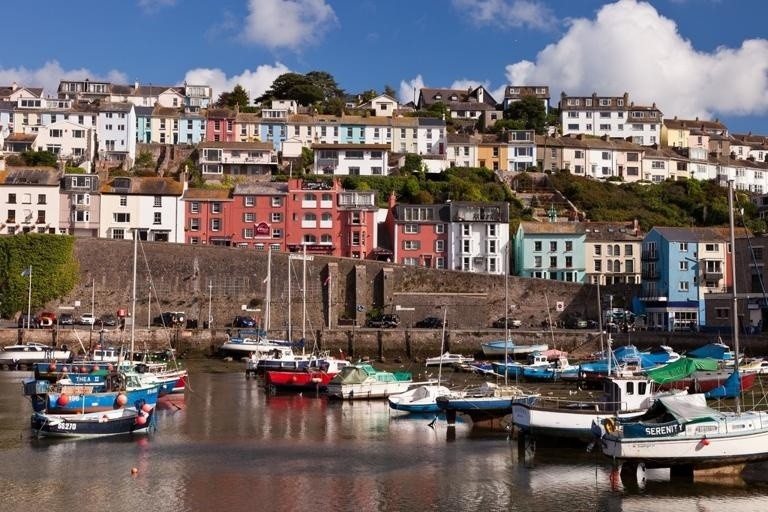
[455,177,767,400]
[434,245,543,411]
[523,456,768,512]
[386,307,469,413]
[218,244,353,388]
[1,223,192,449]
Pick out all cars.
[493,317,522,329]
[416,317,448,329]
[367,313,401,329]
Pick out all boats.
[586,391,768,469]
[508,351,690,449]
[325,362,413,402]
[425,351,476,366]
